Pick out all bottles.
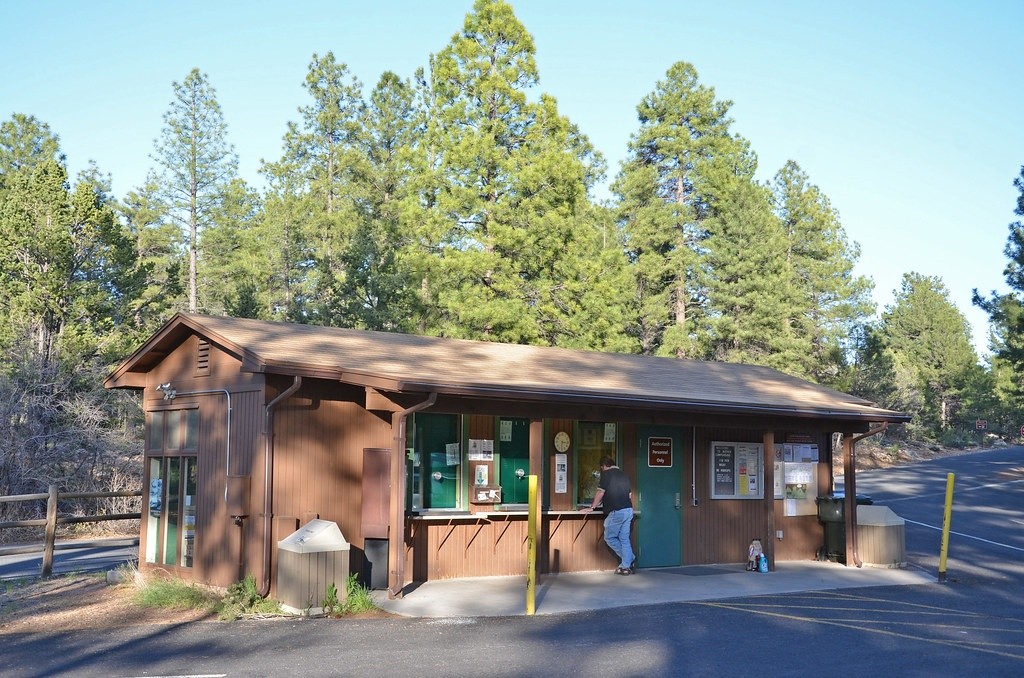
[759,553,769,573]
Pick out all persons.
[580,457,637,576]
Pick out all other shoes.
[614,567,630,576]
[630,557,637,574]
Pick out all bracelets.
[591,506,594,509]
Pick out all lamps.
[156,383,177,400]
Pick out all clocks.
[554,431,571,453]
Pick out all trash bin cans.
[815,493,873,564]
